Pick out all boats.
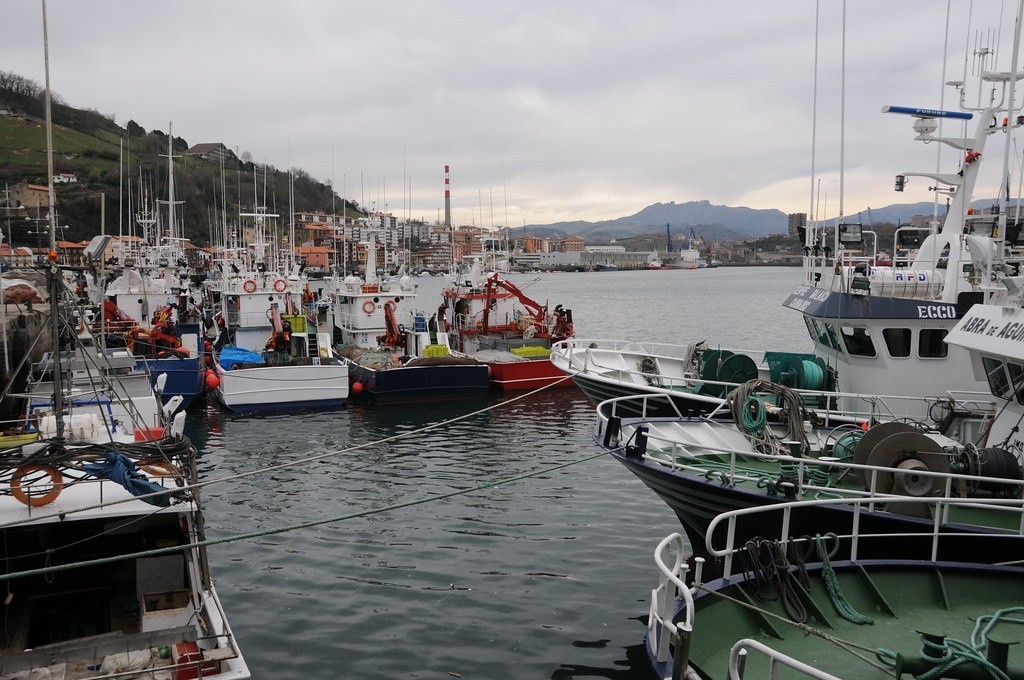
[639,498,1024,680]
[593,245,1024,583]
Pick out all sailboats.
[547,0,1024,416]
[1,0,705,680]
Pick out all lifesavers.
[274,279,286,292]
[8,464,64,508]
[243,279,256,293]
[362,301,375,314]
[384,300,397,312]
